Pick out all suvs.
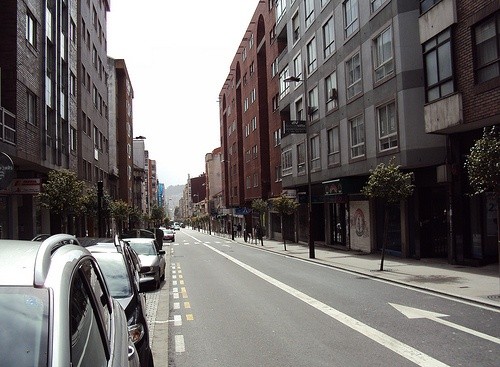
[0,232,141,367]
[30,233,155,367]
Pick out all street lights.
[202,183,211,236]
[194,194,200,232]
[131,136,146,215]
[283,76,315,260]
[221,160,235,240]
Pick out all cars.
[171,222,184,231]
[162,230,175,242]
[121,238,167,290]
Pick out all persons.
[233,222,264,244]
